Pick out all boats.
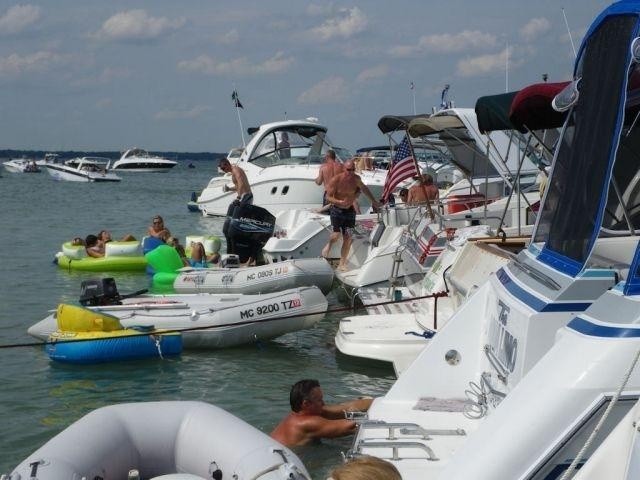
[107,146,177,173]
[29,284,329,348]
[52,232,222,270]
[44,304,182,364]
[45,156,121,186]
[6,399,311,480]
[3,151,63,174]
[145,245,332,293]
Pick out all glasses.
[346,169,355,171]
[153,222,160,224]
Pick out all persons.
[399,188,410,203]
[308,185,361,215]
[71,214,220,268]
[406,173,439,205]
[315,150,343,215]
[267,378,375,446]
[25,159,37,173]
[325,455,403,480]
[321,159,379,270]
[217,156,255,266]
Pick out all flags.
[231,90,244,111]
[380,133,418,206]
